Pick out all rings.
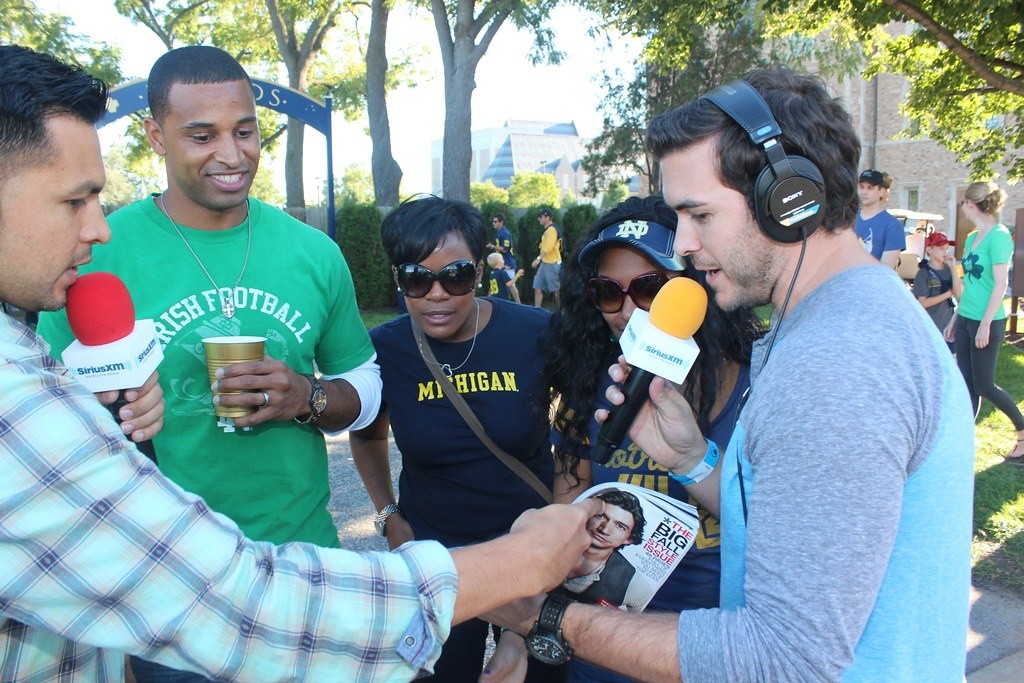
[260,391,269,408]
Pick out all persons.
[350,198,565,683]
[472,70,975,683]
[486,215,520,303]
[486,253,525,300]
[0,41,604,683]
[557,491,647,606]
[943,181,1024,459]
[478,197,769,683]
[34,45,382,683]
[532,209,562,309]
[913,232,962,353]
[904,220,935,259]
[852,169,906,270]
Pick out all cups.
[202,336,268,418]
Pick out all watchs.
[294,373,327,425]
[374,504,401,537]
[525,593,579,666]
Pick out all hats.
[536,208,552,217]
[925,232,957,247]
[578,219,690,271]
[858,169,884,186]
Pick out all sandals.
[1006,439,1024,459]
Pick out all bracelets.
[668,438,720,485]
[537,256,541,259]
[516,274,519,277]
[490,245,494,249]
[500,627,525,639]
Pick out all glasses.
[392,260,480,298]
[961,200,968,204]
[588,272,670,314]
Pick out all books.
[547,482,700,613]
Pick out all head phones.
[699,80,826,244]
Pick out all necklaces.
[419,299,480,377]
[160,189,251,318]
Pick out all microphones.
[589,277,709,466]
[61,271,165,467]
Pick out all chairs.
[896,253,922,285]
